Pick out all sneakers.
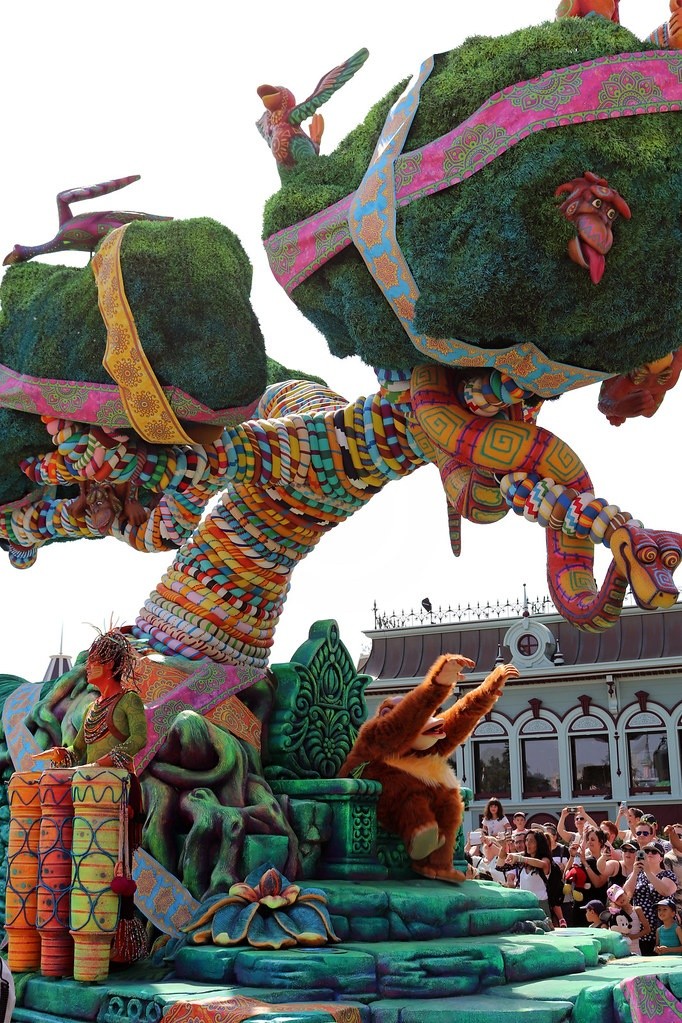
[559,918,567,928]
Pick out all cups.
[500,831,505,841]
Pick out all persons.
[463,798,682,955]
[32,612,148,974]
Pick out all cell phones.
[575,835,582,844]
[637,851,645,868]
[622,801,626,807]
[469,832,482,845]
[605,844,612,856]
[507,827,512,835]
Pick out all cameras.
[567,808,579,813]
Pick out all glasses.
[575,817,584,821]
[622,848,637,853]
[677,833,682,839]
[513,840,524,845]
[644,849,662,857]
[635,831,650,836]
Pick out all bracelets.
[570,854,575,857]
[488,842,494,848]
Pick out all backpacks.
[518,854,565,906]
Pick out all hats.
[641,814,657,826]
[642,842,665,854]
[580,900,604,914]
[531,823,547,832]
[620,840,640,851]
[607,884,624,902]
[514,812,525,817]
[653,899,676,910]
